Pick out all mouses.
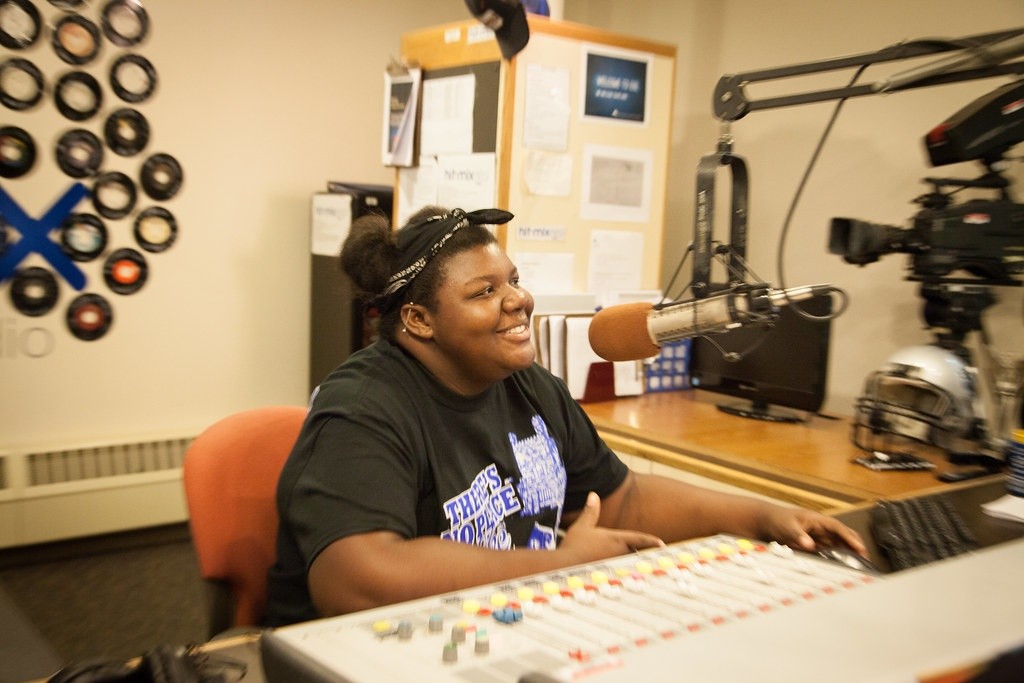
[803,534,882,577]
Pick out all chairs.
[182,406,309,645]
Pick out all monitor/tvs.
[688,294,833,424]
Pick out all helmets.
[852,345,985,466]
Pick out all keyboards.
[868,494,981,574]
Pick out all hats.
[465,0,530,59]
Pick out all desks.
[580,388,951,511]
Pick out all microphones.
[589,283,834,364]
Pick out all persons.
[253,206,866,629]
